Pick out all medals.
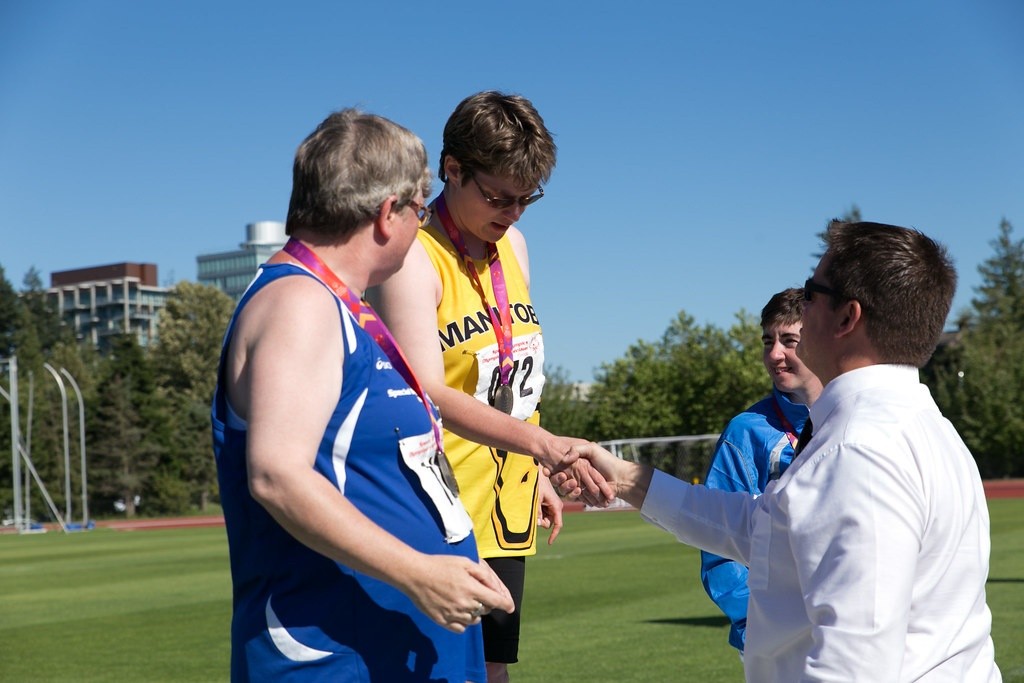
[496,385,512,416]
[437,449,460,500]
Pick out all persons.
[701,288,825,663]
[365,91,614,683]
[541,218,1004,683]
[211,108,516,683]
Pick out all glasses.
[471,177,546,211]
[801,278,851,314]
[404,194,436,232]
